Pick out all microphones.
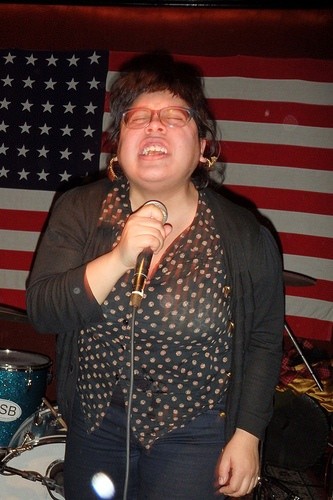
[130,200,169,308]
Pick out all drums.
[0,406,67,500]
[0,348,53,457]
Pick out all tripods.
[226,436,303,500]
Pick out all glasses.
[120,105,196,129]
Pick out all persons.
[25,49,284,500]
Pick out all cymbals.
[281,268,316,287]
[0,305,29,321]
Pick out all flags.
[0,45,333,339]
